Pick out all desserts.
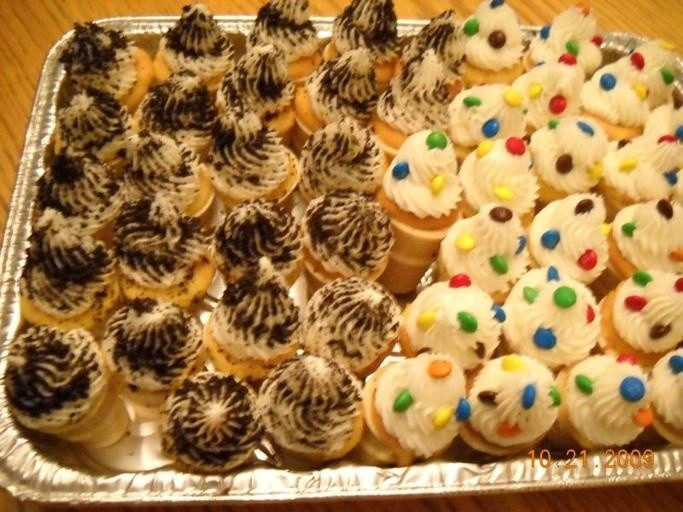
[1,0,683,474]
